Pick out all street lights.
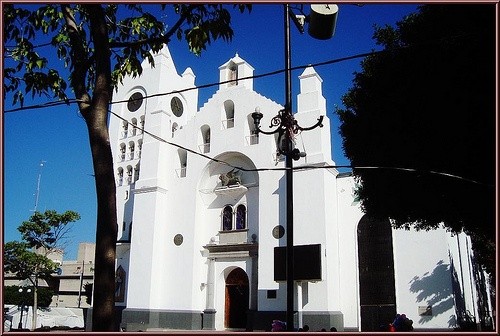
[252,2,324,330]
